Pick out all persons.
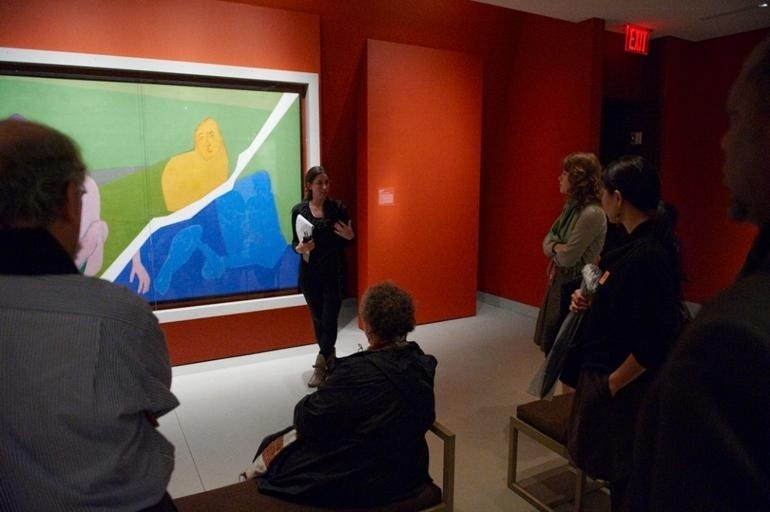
[559,154,691,512]
[0,119,179,512]
[291,166,356,386]
[257,281,438,507]
[533,152,608,396]
[610,38,770,512]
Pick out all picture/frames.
[0,46,320,325]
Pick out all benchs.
[506,393,608,511]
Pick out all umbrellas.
[540,263,611,401]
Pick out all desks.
[174,388,458,508]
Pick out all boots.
[308,348,335,386]
